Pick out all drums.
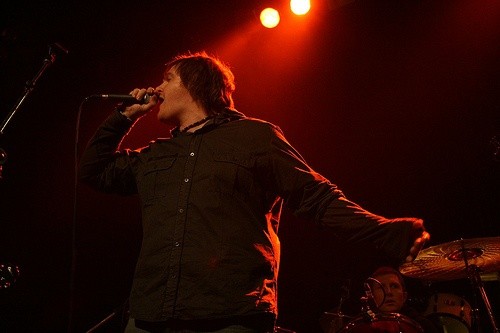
[418,292,479,333]
[334,311,425,333]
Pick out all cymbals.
[399,236,500,282]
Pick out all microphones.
[90,93,151,104]
[364,282,381,314]
[48,41,72,61]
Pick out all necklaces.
[173,115,213,132]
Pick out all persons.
[75,52,436,333]
